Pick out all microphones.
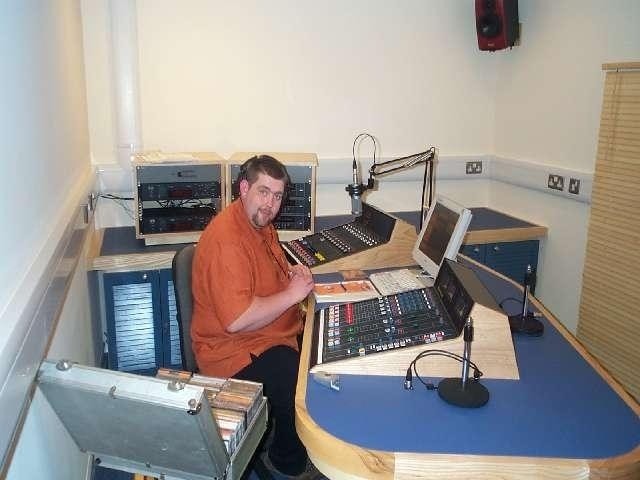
[351,160,364,218]
[403,370,412,390]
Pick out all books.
[155,367,264,459]
[312,280,377,299]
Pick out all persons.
[190,155,316,477]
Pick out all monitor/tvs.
[410,194,473,287]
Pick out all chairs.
[172,244,278,479]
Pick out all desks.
[87,207,640,479]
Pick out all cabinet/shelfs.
[103,255,184,376]
[459,239,539,297]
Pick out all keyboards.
[368,269,425,297]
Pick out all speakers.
[474,0,520,52]
